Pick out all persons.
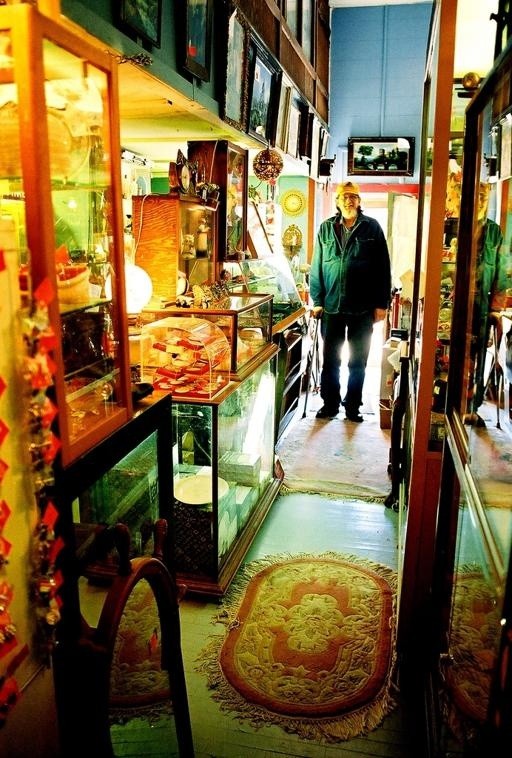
[309,181,391,422]
[464,182,507,427]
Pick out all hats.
[336,181,360,195]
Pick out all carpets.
[104,585,167,726]
[197,548,402,746]
[443,571,504,738]
[276,412,395,506]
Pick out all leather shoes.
[316,405,338,418]
[346,410,363,422]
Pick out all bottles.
[122,174,142,195]
[182,234,196,261]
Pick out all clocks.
[175,160,191,197]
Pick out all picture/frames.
[348,136,416,179]
[222,5,252,131]
[248,48,278,148]
[235,201,272,258]
[109,3,174,48]
[175,1,215,82]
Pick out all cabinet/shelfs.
[176,349,286,587]
[22,7,132,467]
[438,71,511,756]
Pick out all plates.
[173,474,229,506]
